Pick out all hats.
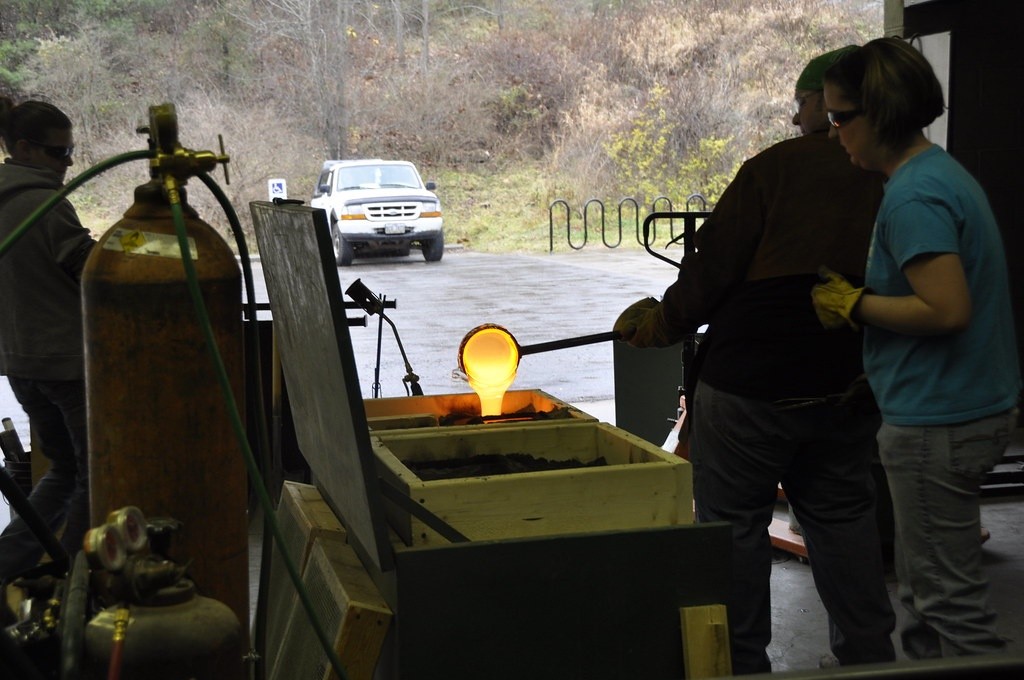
[795,45,862,90]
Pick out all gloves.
[810,264,865,333]
[613,297,672,349]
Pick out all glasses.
[827,109,862,129]
[795,89,824,106]
[25,138,74,160]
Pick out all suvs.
[310,158,445,266]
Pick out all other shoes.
[818,654,844,670]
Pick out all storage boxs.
[244,197,731,679]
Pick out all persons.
[615,45,897,675]
[810,38,1018,660]
[0,97,99,581]
